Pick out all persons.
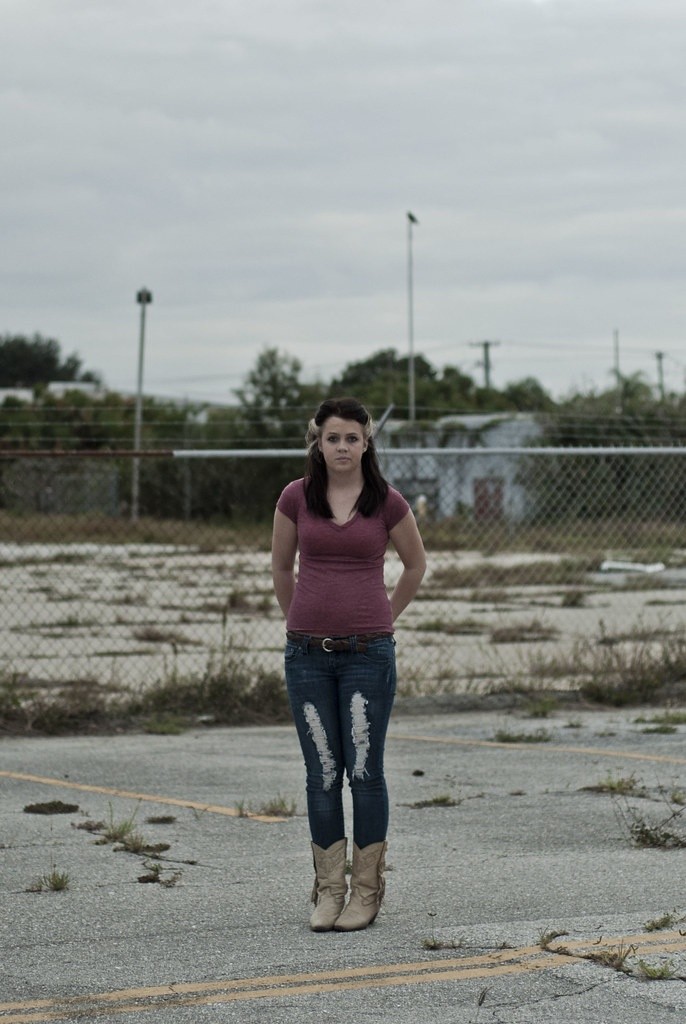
[272,398,427,931]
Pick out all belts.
[288,632,387,652]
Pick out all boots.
[310,838,348,930]
[335,837,387,930]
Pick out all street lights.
[655,351,665,399]
[130,286,153,521]
